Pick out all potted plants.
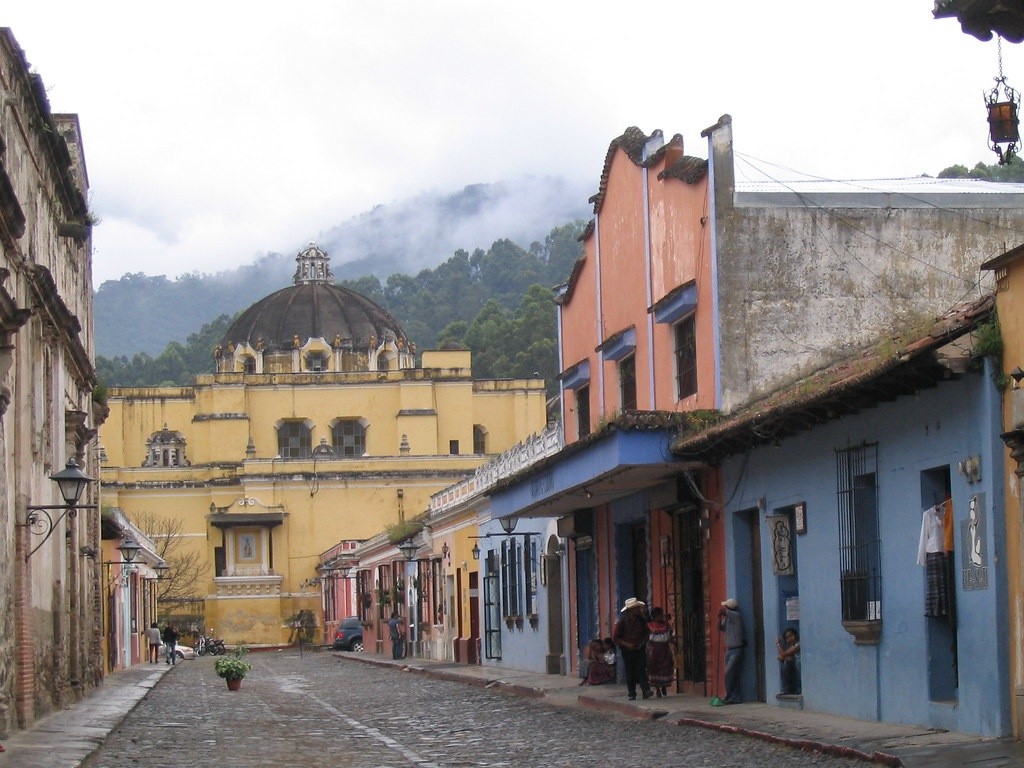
[57,211,103,238]
[383,518,424,545]
[215,655,253,690]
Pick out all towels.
[945,499,955,551]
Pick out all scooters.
[194,627,225,657]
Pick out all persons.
[579,638,616,686]
[162,621,181,665]
[776,627,801,694]
[388,612,402,660]
[647,606,676,698]
[717,598,748,704]
[613,598,655,701]
[144,622,163,664]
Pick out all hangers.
[935,498,953,514]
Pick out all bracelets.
[777,644,780,646]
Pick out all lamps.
[468,536,489,560]
[487,518,542,537]
[338,561,364,579]
[399,538,437,563]
[146,560,172,585]
[442,542,450,559]
[583,488,593,499]
[27,457,98,561]
[103,536,148,589]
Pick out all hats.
[621,598,646,613]
[721,599,738,610]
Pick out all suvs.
[333,616,364,653]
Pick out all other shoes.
[643,691,654,699]
[629,695,635,701]
[723,697,741,704]
[166,661,170,665]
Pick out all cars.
[158,640,196,661]
